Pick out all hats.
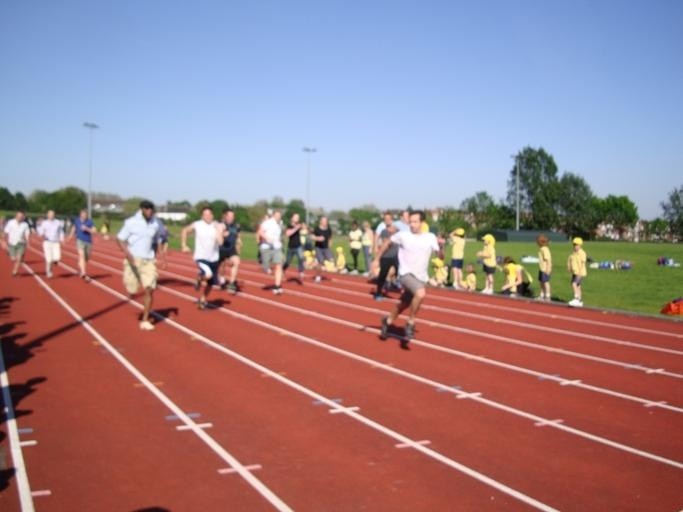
[572,237,584,246]
[431,257,443,267]
[453,227,465,237]
[335,246,342,253]
[481,233,494,241]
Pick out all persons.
[34,211,68,278]
[334,246,346,274]
[567,237,586,306]
[370,209,445,340]
[429,231,450,288]
[181,208,225,312]
[300,215,346,274]
[115,201,171,330]
[393,211,409,231]
[534,234,552,304]
[255,208,287,294]
[69,210,98,284]
[373,212,401,302]
[218,208,242,291]
[283,212,305,279]
[360,221,374,279]
[475,234,498,295]
[0,211,39,277]
[347,219,363,275]
[499,256,533,299]
[448,228,467,292]
[462,262,477,293]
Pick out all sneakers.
[567,297,584,308]
[395,279,402,289]
[372,293,381,300]
[405,323,416,339]
[449,283,458,291]
[198,299,208,310]
[534,296,544,303]
[545,297,551,303]
[195,278,202,291]
[480,289,493,295]
[226,283,237,293]
[380,315,391,338]
[273,284,283,296]
[139,320,155,331]
